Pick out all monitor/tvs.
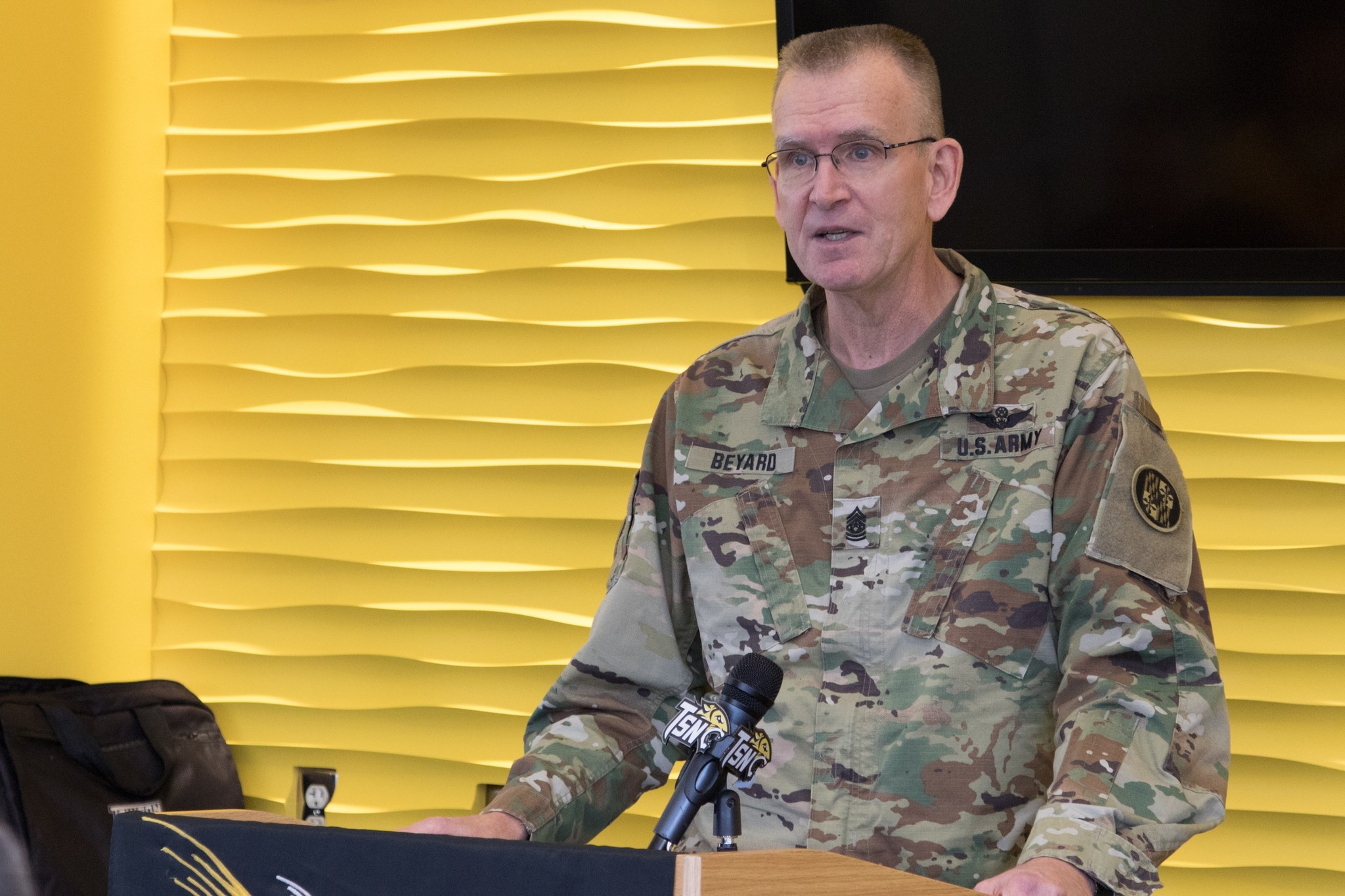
[777,1,1345,297]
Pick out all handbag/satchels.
[0,675,246,896]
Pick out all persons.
[398,25,1231,896]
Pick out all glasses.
[761,137,937,185]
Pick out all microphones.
[647,652,783,850]
